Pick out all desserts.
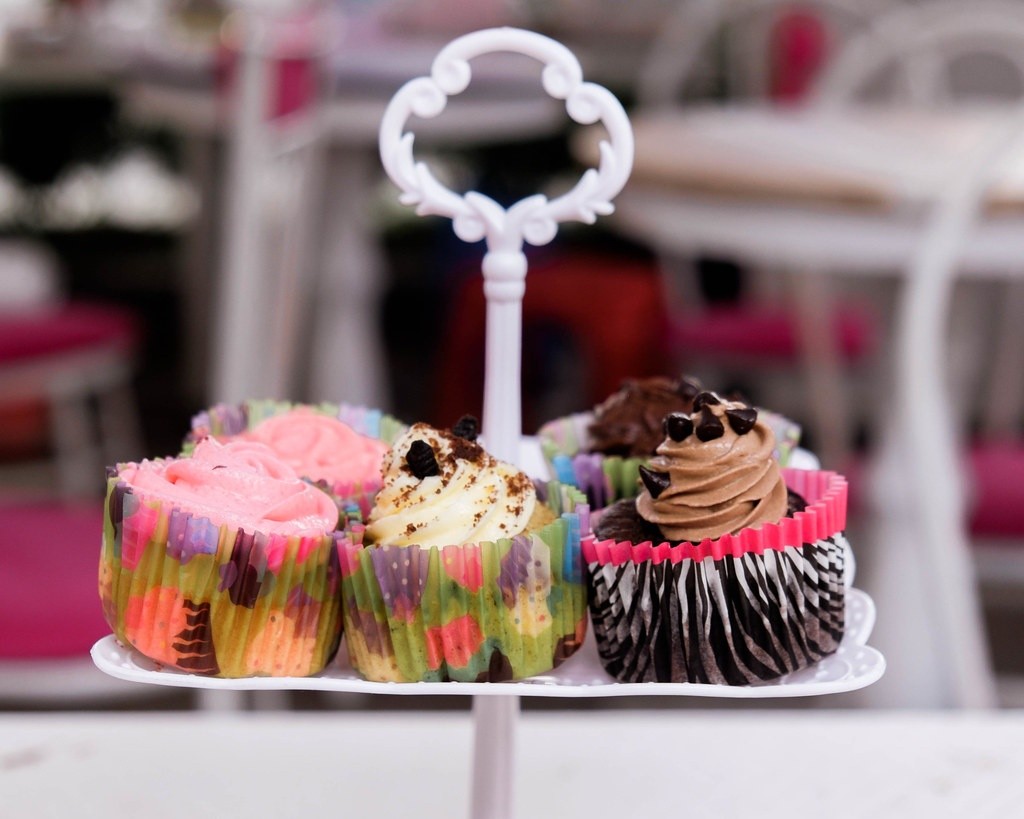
[95,373,853,689]
